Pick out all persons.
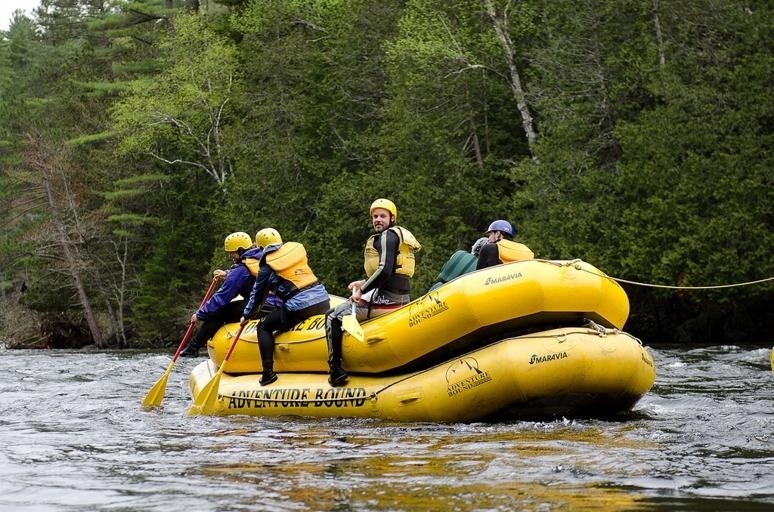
[428,237,490,293]
[325,199,422,387]
[179,232,273,358]
[476,220,534,271]
[240,228,330,387]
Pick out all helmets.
[369,196,398,223]
[223,230,254,252]
[254,226,283,250]
[483,218,514,236]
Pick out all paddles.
[186,319,250,417]
[341,286,364,343]
[141,275,218,408]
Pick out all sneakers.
[326,368,351,387]
[256,369,279,387]
[178,341,203,360]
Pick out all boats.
[208,258,635,372]
[184,326,656,421]
